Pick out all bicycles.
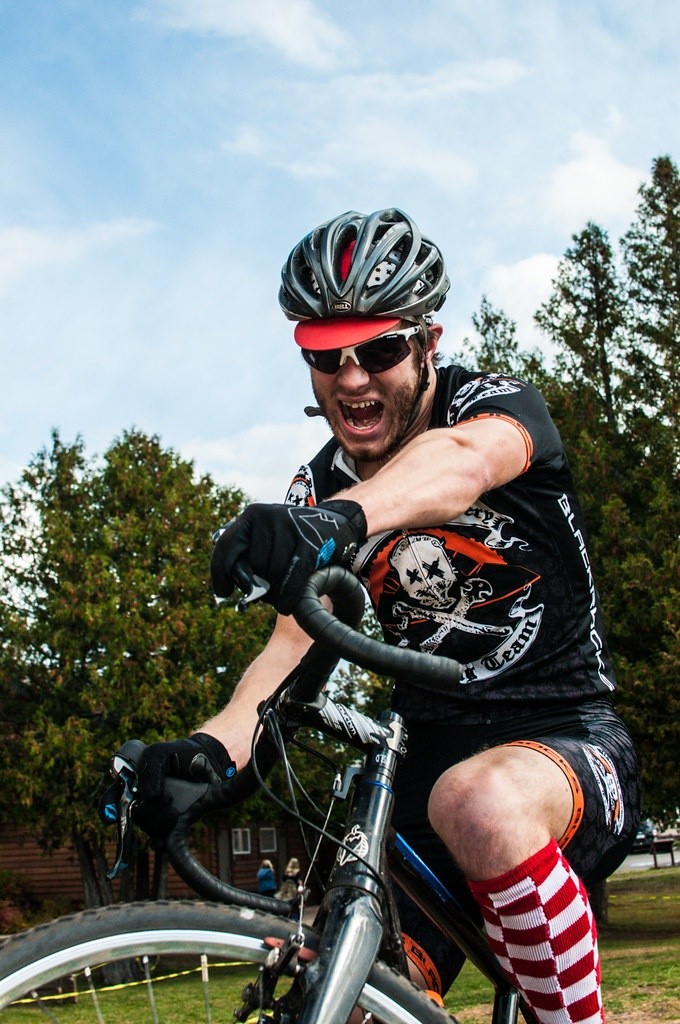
[1,546,584,1024]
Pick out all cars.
[632,816,658,854]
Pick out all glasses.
[301,324,422,375]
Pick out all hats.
[294,237,427,352]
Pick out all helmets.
[278,208,452,321]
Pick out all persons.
[280,855,305,921]
[126,205,651,1024]
[256,856,277,898]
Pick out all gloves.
[99,732,237,881]
[211,498,368,616]
